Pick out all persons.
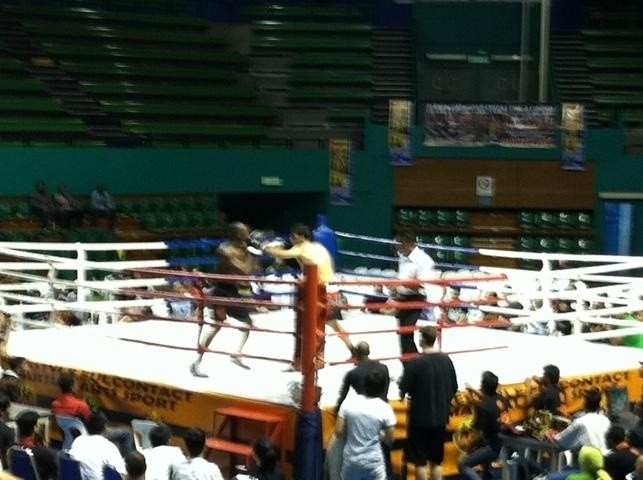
[1,181,642,479]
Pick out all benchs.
[569,0,643,164]
[0,0,377,154]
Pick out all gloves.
[273,237,288,247]
[247,230,265,249]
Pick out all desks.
[1,399,52,448]
[495,414,577,480]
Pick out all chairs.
[52,413,87,448]
[97,460,126,479]
[50,450,93,479]
[0,197,236,289]
[127,420,165,453]
[4,444,43,479]
[392,208,600,274]
